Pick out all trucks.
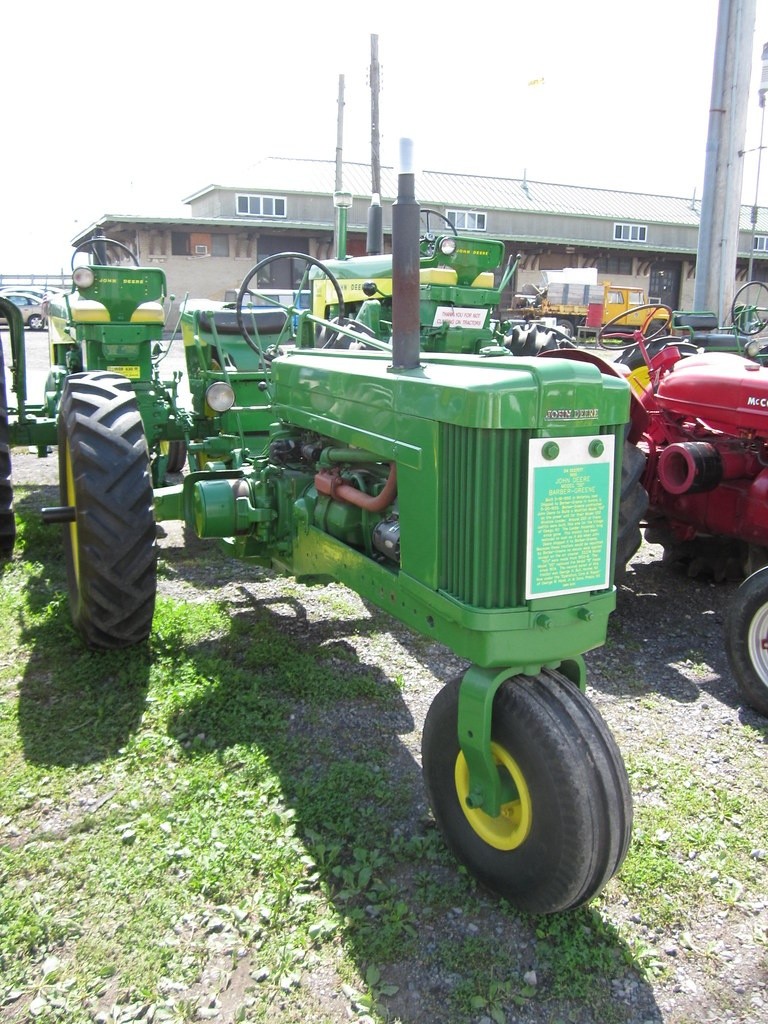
[499,282,672,341]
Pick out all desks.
[576,325,605,350]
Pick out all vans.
[222,289,311,332]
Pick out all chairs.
[671,310,748,353]
[193,309,294,382]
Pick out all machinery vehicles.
[0,172,767,916]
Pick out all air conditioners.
[195,246,207,254]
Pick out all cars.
[0,292,46,331]
[0,288,45,298]
